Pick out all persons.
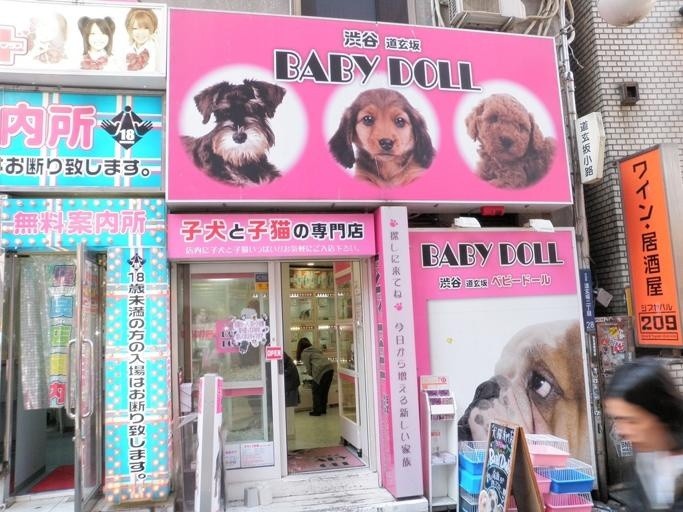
[125,8,158,71]
[265,351,300,441]
[78,17,115,70]
[601,360,683,512]
[296,337,334,416]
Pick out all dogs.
[179,78,287,189]
[327,86,437,188]
[463,91,555,192]
[455,317,593,465]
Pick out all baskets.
[459,432,594,510]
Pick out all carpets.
[284,448,367,476]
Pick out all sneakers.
[309,409,327,416]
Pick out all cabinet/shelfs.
[421,385,461,511]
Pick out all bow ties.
[127,49,149,71]
[84,55,106,70]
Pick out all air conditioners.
[448,0,529,29]
